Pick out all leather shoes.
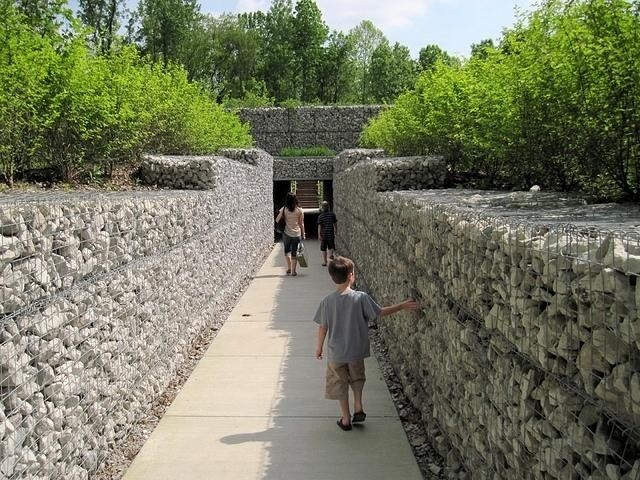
[286,270,291,276]
[292,272,297,276]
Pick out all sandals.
[329,255,334,259]
[322,262,327,266]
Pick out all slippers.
[352,411,366,425]
[337,417,352,431]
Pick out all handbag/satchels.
[297,239,308,267]
[275,215,286,234]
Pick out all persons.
[317,200,339,267]
[275,192,306,276]
[312,255,420,431]
